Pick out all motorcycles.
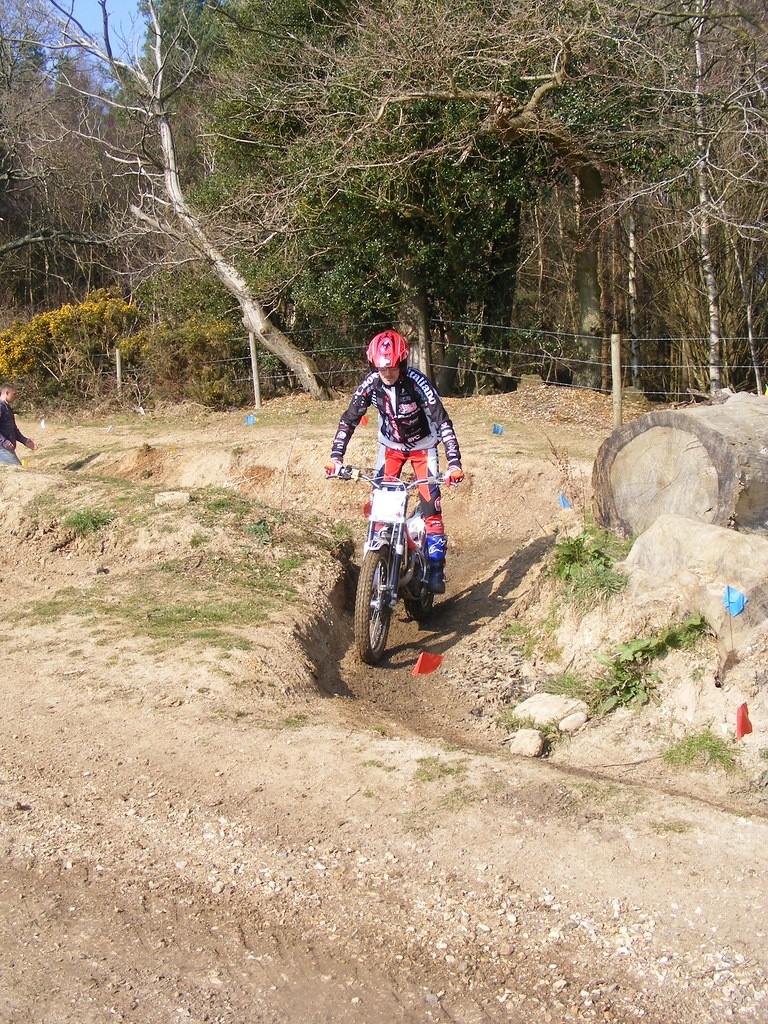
[325,464,458,665]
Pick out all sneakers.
[428,559,446,594]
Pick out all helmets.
[366,331,410,367]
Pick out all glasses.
[378,367,400,371]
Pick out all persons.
[0,383,37,465]
[324,332,465,593]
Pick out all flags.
[246,415,255,425]
[493,422,504,435]
[723,587,749,617]
[737,702,753,738]
[411,651,444,678]
[360,415,368,427]
[560,495,570,508]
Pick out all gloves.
[323,458,343,475]
[445,468,464,488]
